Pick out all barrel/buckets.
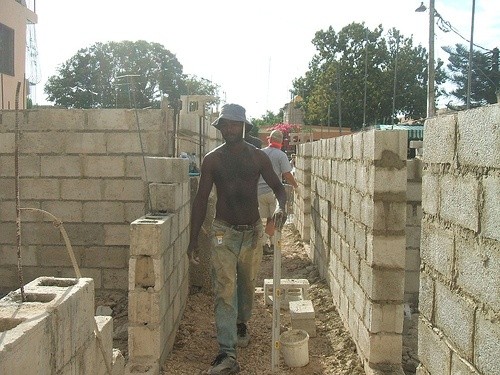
[280,329,311,368]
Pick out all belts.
[214,218,262,231]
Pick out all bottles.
[188,152,197,173]
[180,152,188,159]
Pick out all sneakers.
[207,353,240,374]
[236,321,251,348]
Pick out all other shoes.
[269,244,274,252]
[263,243,273,253]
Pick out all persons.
[187,103,287,374]
[257,130,301,253]
[290,153,296,176]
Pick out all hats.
[212,104,254,134]
[266,130,284,142]
